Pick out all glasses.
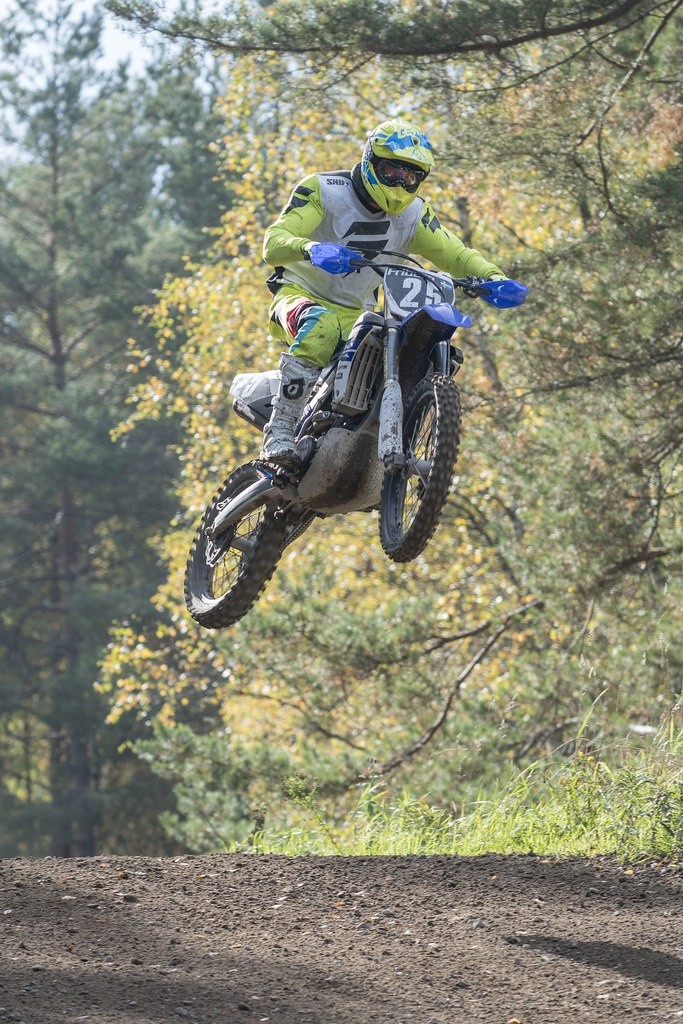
[373,158,425,190]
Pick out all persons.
[262,119,509,467]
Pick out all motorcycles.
[179,251,524,634]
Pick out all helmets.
[360,120,435,216]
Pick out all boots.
[259,354,326,473]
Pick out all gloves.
[488,272,509,282]
[302,241,320,260]
